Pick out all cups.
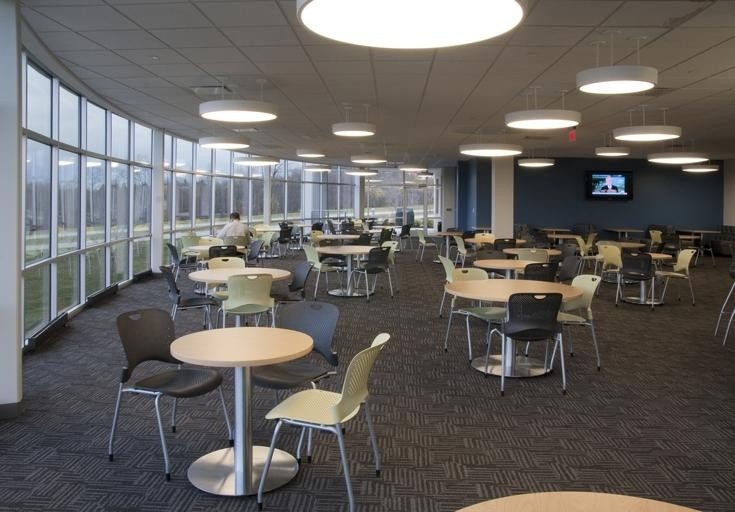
[326,230,330,236]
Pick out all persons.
[600,176,618,193]
[217,212,250,250]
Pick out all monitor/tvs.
[584,169,634,202]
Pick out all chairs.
[109,308,233,483]
[234,301,340,463]
[257,332,391,512]
[159,218,412,329]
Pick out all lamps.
[295,0,528,50]
[680,164,719,173]
[595,131,631,157]
[517,144,555,167]
[575,30,658,95]
[459,128,523,157]
[199,78,434,183]
[505,85,582,130]
[647,140,710,164]
[613,104,682,142]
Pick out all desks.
[453,491,702,512]
[170,326,314,497]
